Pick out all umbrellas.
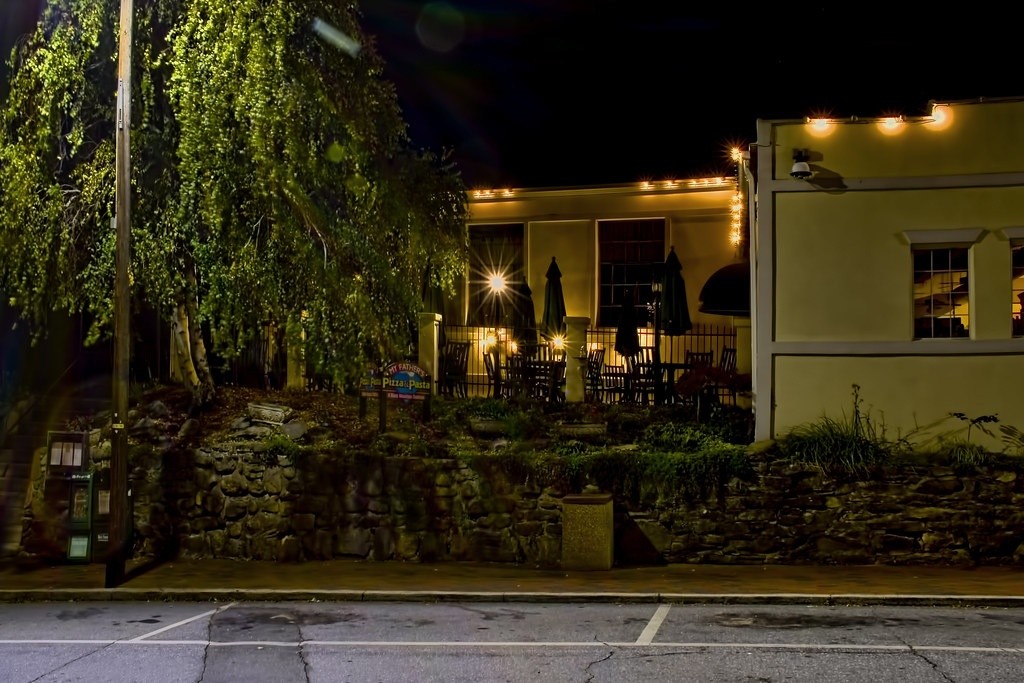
[659,245,693,364]
[541,256,567,361]
[614,287,640,373]
[511,276,538,361]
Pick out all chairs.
[438,340,737,417]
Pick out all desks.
[526,361,566,398]
[639,363,698,404]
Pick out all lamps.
[651,268,663,297]
[789,148,813,180]
